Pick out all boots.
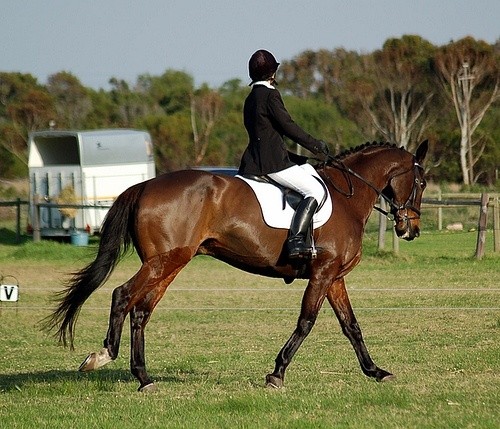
[287,197,318,257]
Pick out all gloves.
[318,140,330,155]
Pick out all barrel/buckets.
[71,233,88,245]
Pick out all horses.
[35,138,429,392]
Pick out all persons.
[238,50,329,261]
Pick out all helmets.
[249,50,280,81]
